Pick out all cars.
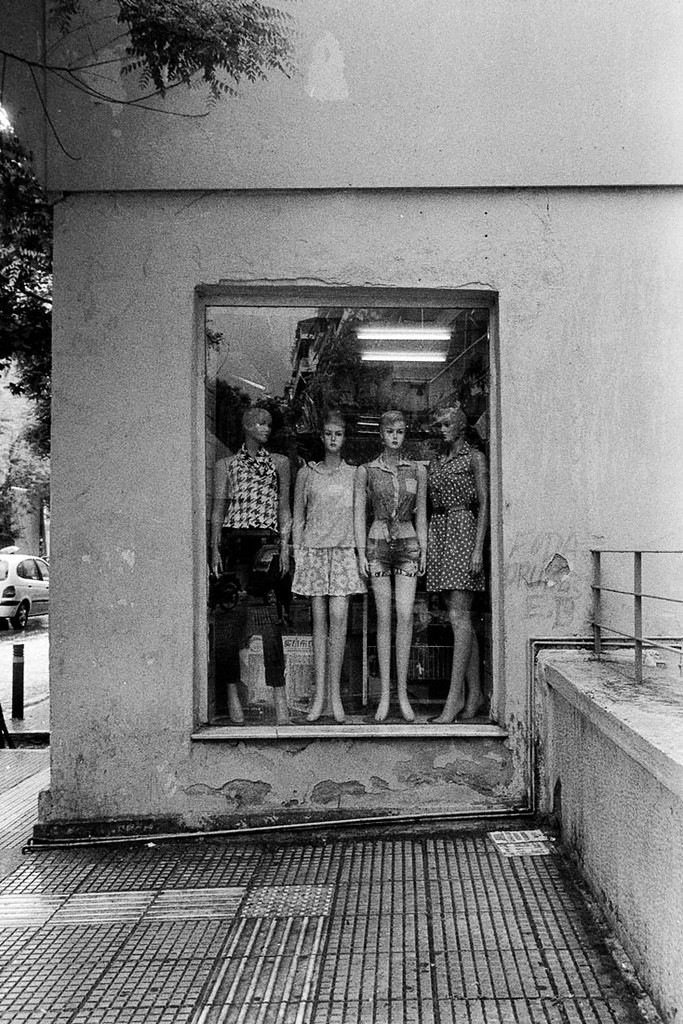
[0,554,50,629]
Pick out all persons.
[290,418,364,723]
[426,407,492,723]
[207,406,294,725]
[352,410,428,722]
